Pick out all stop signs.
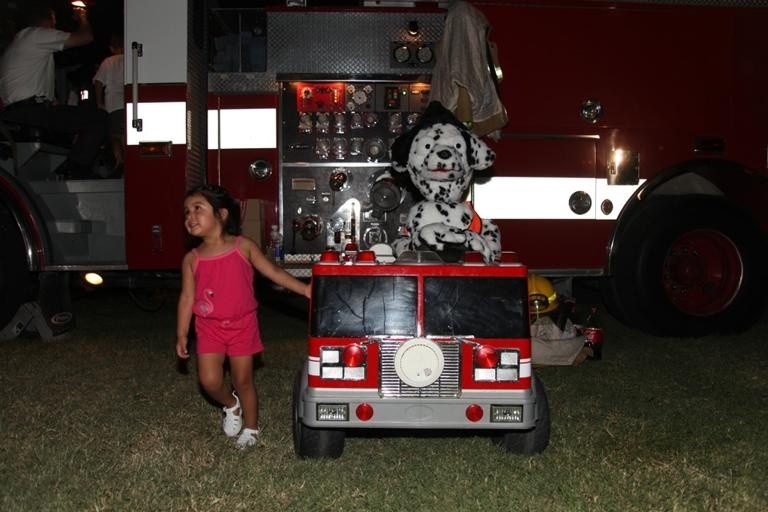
[0,0,768,349]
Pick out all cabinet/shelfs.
[582,307,603,361]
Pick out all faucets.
[234,425,259,450]
[222,390,243,437]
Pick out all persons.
[94,29,124,169]
[1,0,109,181]
[175,184,311,451]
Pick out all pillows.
[527,273,560,315]
